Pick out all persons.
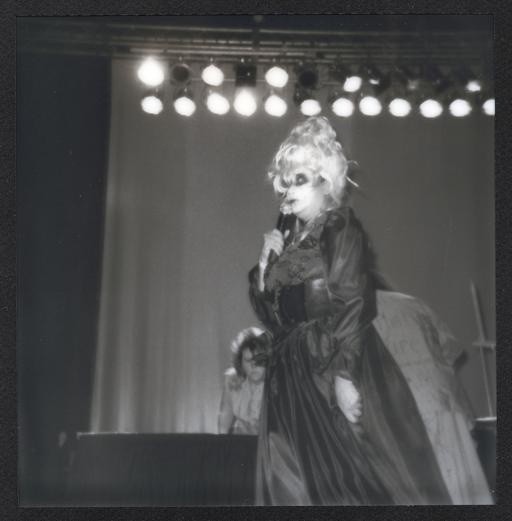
[216,326,269,435]
[246,114,453,505]
[368,270,496,505]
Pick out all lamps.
[136,55,495,121]
